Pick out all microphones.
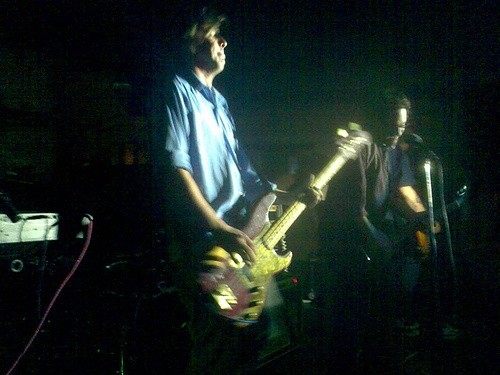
[405,133,440,162]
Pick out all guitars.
[196,122,373,328]
[365,184,469,264]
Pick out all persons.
[312,87,438,341]
[137,8,325,373]
